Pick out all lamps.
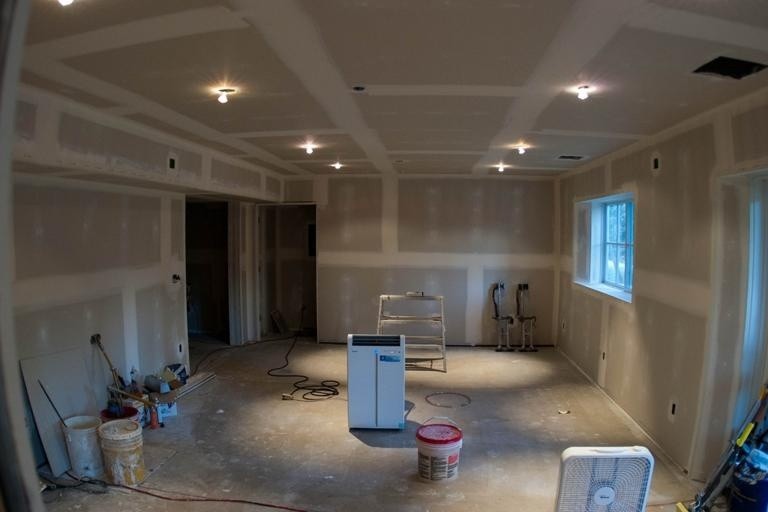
[577,86,589,100]
[218,89,235,104]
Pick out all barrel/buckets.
[97,419,146,487]
[415,414,463,486]
[63,417,101,479]
[100,406,140,426]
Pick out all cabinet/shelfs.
[377,291,447,373]
